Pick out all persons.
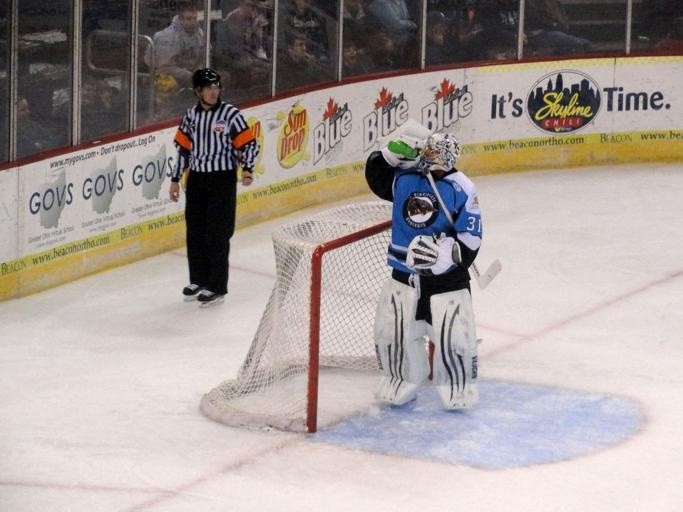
[169,68,262,302]
[364,117,484,413]
[0,0,683,165]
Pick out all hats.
[428,8,456,25]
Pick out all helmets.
[191,67,223,98]
[420,131,463,172]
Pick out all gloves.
[405,233,463,277]
[376,118,431,171]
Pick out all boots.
[196,288,226,303]
[182,283,200,296]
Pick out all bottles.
[387,140,421,159]
